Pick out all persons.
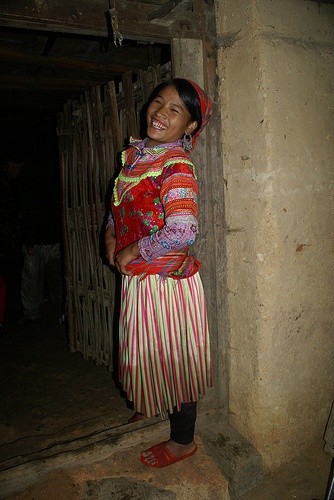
[102,78,215,464]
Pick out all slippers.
[140,441,198,468]
[129,413,157,423]
